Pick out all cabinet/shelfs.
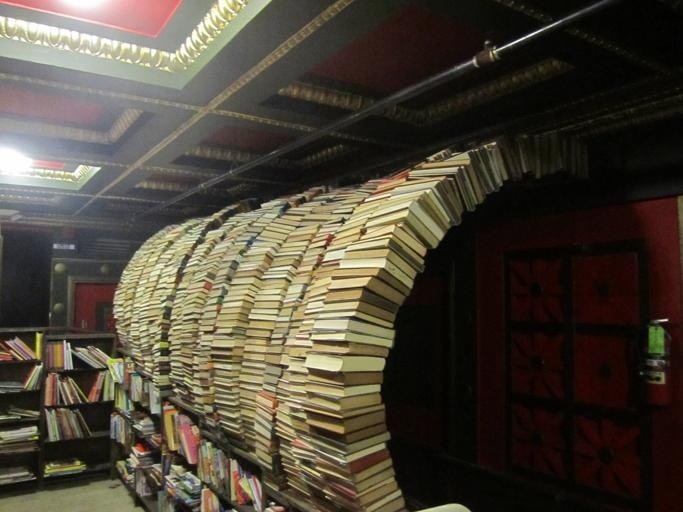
[0,327,49,493]
[41,333,119,495]
[114,365,324,512]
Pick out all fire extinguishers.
[641,317,674,408]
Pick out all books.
[0,330,118,486]
[110,130,591,511]
